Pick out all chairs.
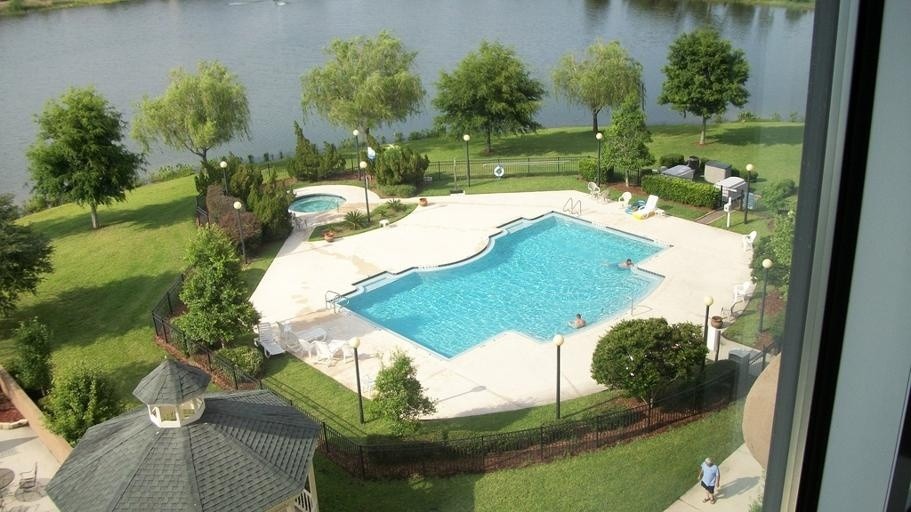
[15,463,44,502]
[588,182,632,208]
[293,216,307,232]
[632,194,659,219]
[254,319,354,367]
[743,230,756,251]
[720,279,758,321]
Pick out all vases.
[712,316,724,329]
[420,198,428,206]
[325,232,335,242]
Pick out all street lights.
[350,337,366,424]
[219,161,229,194]
[359,160,371,221]
[760,259,773,334]
[352,128,361,169]
[233,201,249,264]
[701,297,713,364]
[551,335,566,419]
[744,161,755,224]
[595,133,604,189]
[462,133,471,186]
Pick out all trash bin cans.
[687,155,699,170]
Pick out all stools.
[655,210,665,218]
[379,220,390,231]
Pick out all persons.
[567,313,586,330]
[697,456,720,505]
[600,257,635,273]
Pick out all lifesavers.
[494,166,504,177]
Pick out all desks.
[0,468,15,508]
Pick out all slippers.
[702,496,715,505]
